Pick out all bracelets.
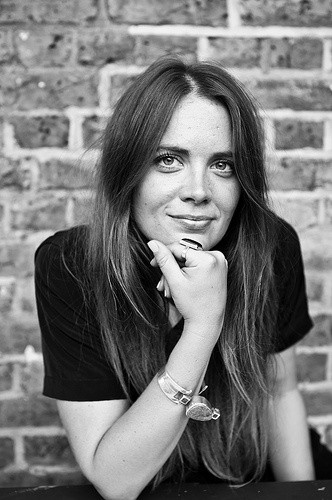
[157,367,220,422]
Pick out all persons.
[34,53,332,500]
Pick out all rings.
[180,238,202,258]
